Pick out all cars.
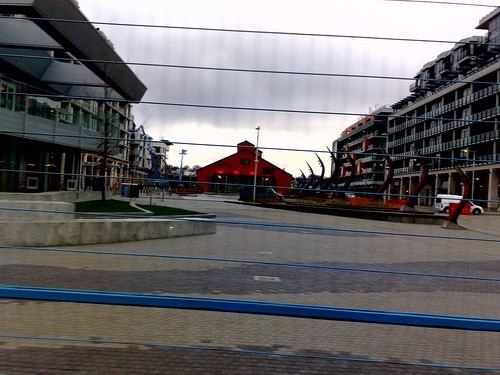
[345,192,356,198]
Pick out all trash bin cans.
[120,182,139,198]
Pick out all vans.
[239,185,284,202]
[433,193,485,215]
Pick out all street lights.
[252,127,260,201]
